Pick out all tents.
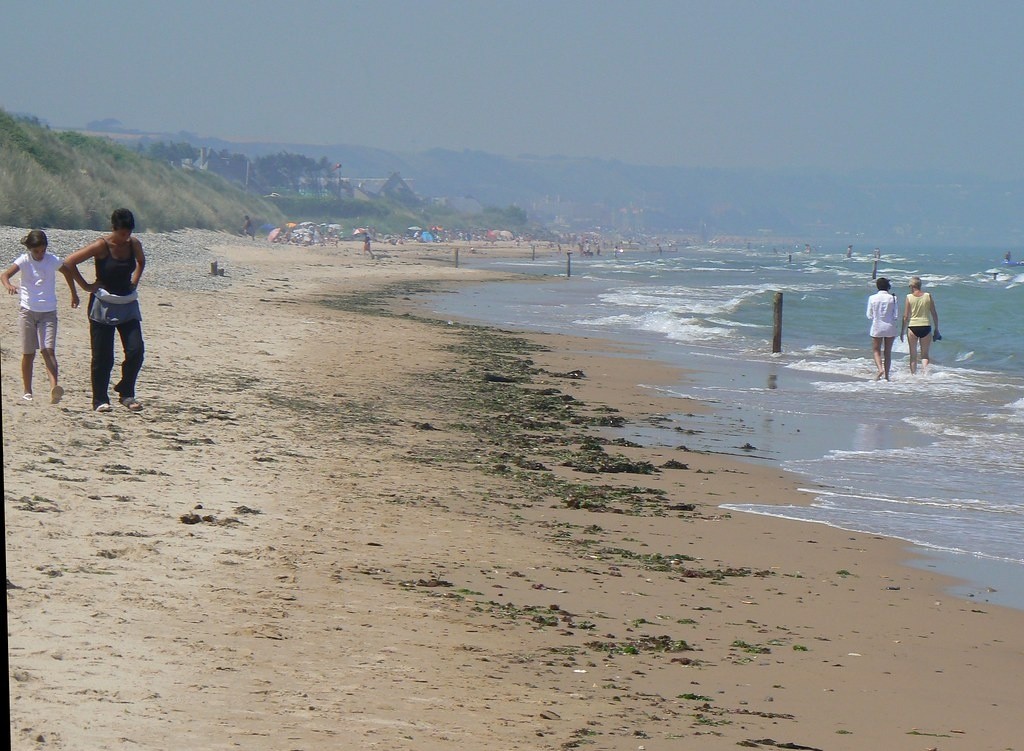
[421,232,433,242]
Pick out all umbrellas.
[263,223,276,230]
[409,225,422,231]
[286,221,342,230]
[268,228,281,240]
[352,228,366,235]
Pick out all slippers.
[118,396,143,410]
[96,404,112,412]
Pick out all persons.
[363,231,375,259]
[900,276,940,376]
[847,245,853,258]
[64,208,145,411]
[456,231,533,247]
[804,244,811,253]
[0,230,80,404]
[284,226,340,247]
[1006,251,1011,261]
[866,278,899,381]
[434,230,453,243]
[242,215,255,241]
[381,233,405,244]
[555,230,624,258]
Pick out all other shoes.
[51,385,64,403]
[23,392,32,401]
